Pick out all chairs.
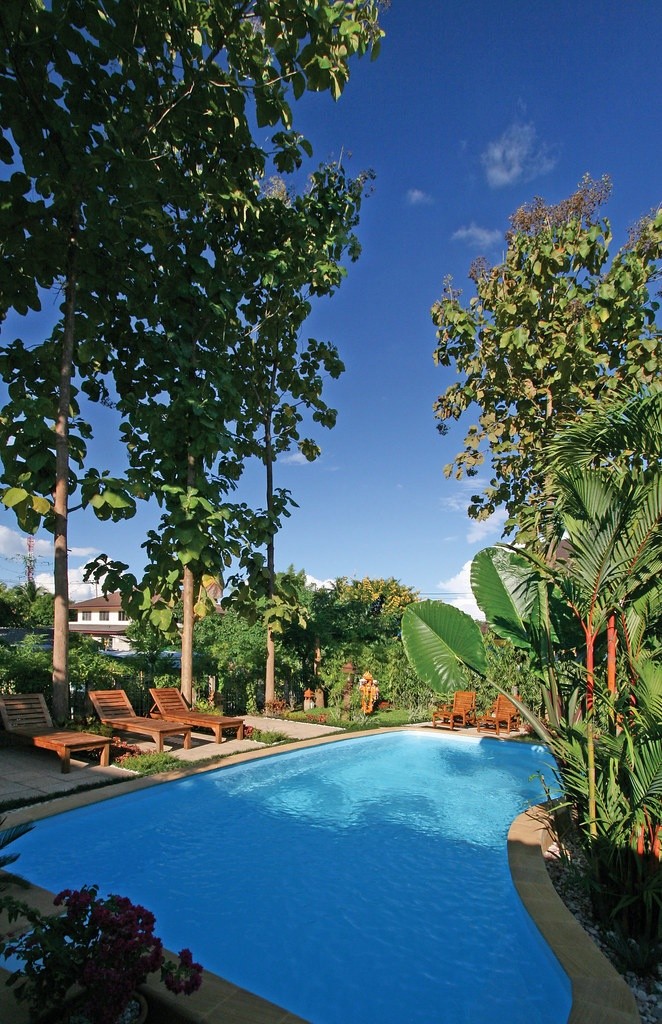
[146,688,244,744]
[0,693,111,773]
[476,695,522,734]
[87,690,192,753]
[433,690,476,730]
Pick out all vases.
[58,989,147,1024]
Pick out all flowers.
[0,884,204,1024]
